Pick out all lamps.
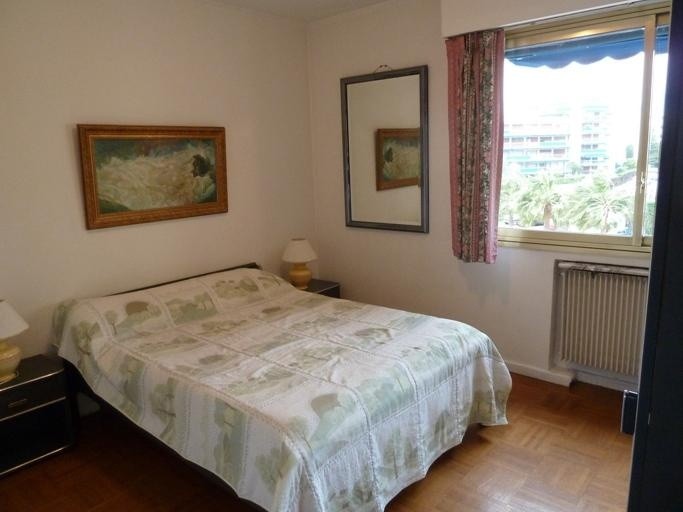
[281,238,319,289]
[0,298,29,383]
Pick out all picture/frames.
[76,123,229,231]
[374,127,421,191]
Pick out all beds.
[56,261,491,511]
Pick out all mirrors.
[339,64,428,234]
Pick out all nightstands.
[0,354,76,475]
[295,278,340,298]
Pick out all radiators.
[553,262,649,384]
